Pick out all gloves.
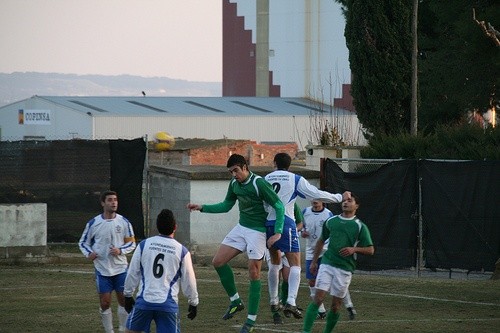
[125,297,135,314]
[188,305,197,319]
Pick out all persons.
[79,190,138,333]
[187,153,285,333]
[265,193,375,333]
[123,208,199,333]
[260,153,351,325]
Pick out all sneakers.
[284,303,304,319]
[297,305,304,312]
[316,311,327,320]
[223,299,244,320]
[348,308,356,320]
[240,324,253,333]
[273,313,284,324]
[271,303,284,312]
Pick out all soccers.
[153,131,175,151]
